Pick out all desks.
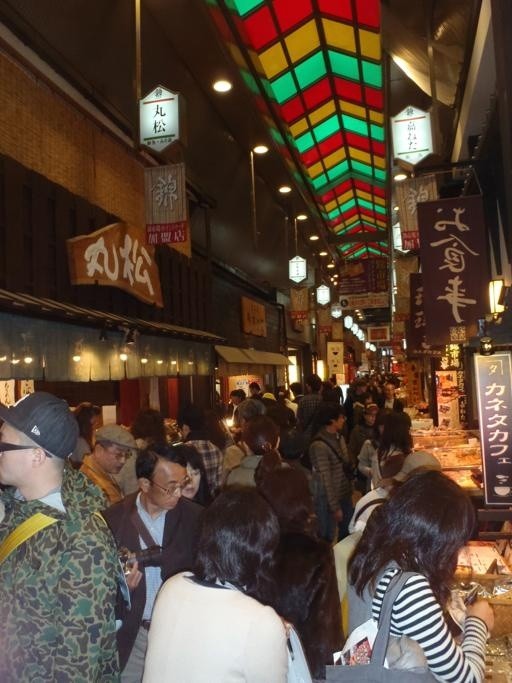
[397,404,511,682]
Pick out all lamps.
[485,276,508,322]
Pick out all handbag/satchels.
[325,571,443,683]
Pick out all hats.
[93,422,140,450]
[391,450,443,483]
[0,391,80,460]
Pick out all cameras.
[118,545,161,575]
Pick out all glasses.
[112,451,133,460]
[151,476,191,497]
